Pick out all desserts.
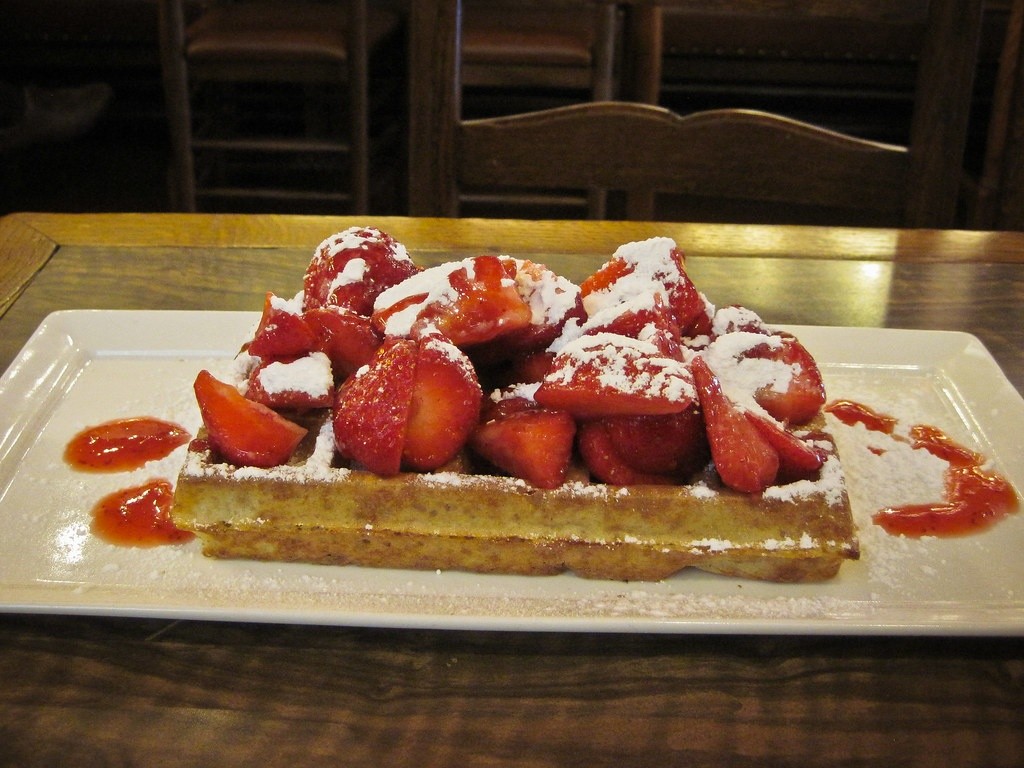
[168,223,863,583]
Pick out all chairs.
[159,1,987,222]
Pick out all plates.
[0,309,1024,634]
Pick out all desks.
[0,211,1023,768]
